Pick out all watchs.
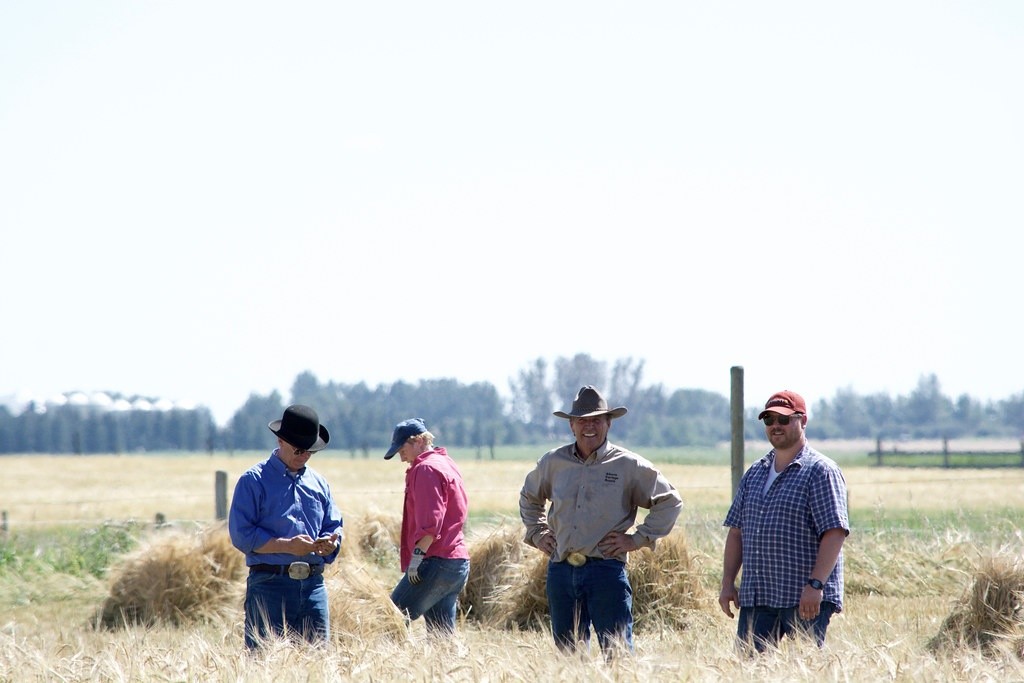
[413,548,426,556]
[807,576,825,590]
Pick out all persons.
[718,390,850,659]
[229,403,344,651]
[383,418,471,635]
[519,385,682,659]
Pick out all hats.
[758,390,806,420]
[552,385,628,420]
[383,417,427,460]
[268,405,330,451]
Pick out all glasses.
[763,414,802,426]
[285,443,317,456]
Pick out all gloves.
[407,555,424,587]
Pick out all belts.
[249,561,324,580]
